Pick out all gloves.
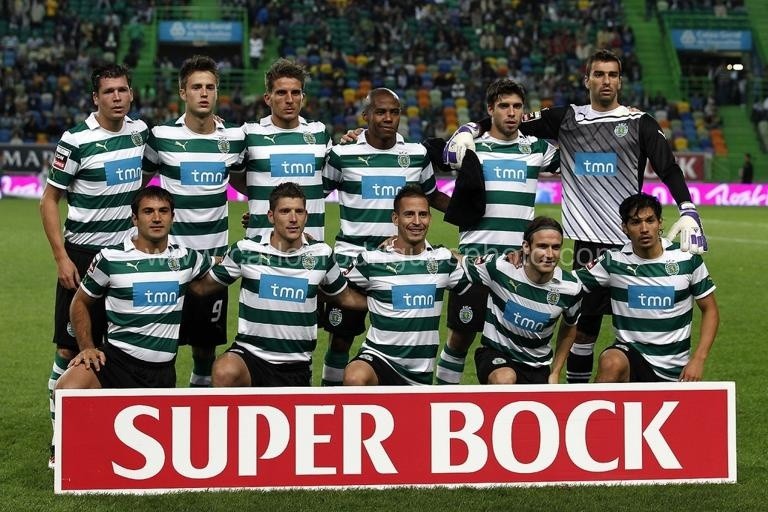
[443,121,480,169]
[666,202,708,255]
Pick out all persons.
[2,1,768,183]
[439,49,710,382]
[142,54,247,387]
[47,185,222,472]
[433,81,559,385]
[229,58,365,329]
[340,184,524,387]
[450,216,586,385]
[37,63,147,435]
[186,183,368,387]
[321,86,452,387]
[506,193,719,383]
